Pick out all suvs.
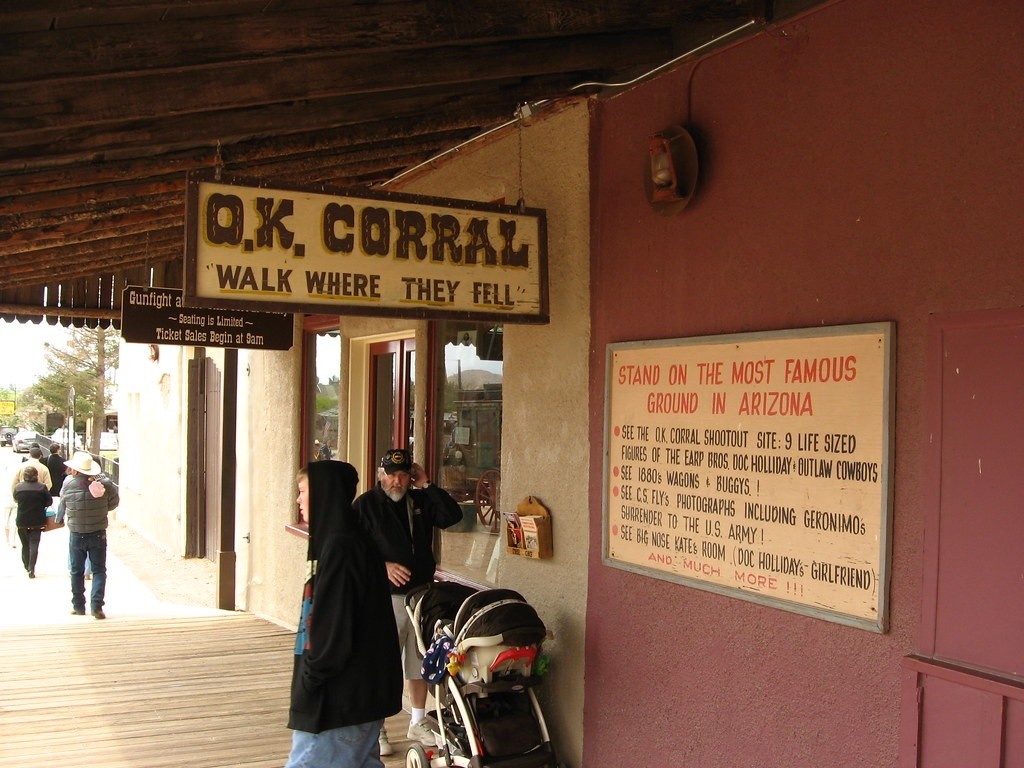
[0,426,19,447]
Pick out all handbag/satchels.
[42,505,65,532]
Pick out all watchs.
[422,480,431,490]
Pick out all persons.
[351,449,464,754]
[283,460,404,768]
[5,442,67,549]
[14,467,53,578]
[55,452,119,619]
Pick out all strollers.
[404,578,559,768]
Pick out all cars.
[12,430,36,453]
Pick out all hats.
[379,448,412,475]
[63,450,101,475]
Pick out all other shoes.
[85,575,90,580]
[90,607,105,618]
[29,570,35,578]
[71,609,85,615]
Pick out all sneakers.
[377,725,393,755]
[407,716,439,746]
